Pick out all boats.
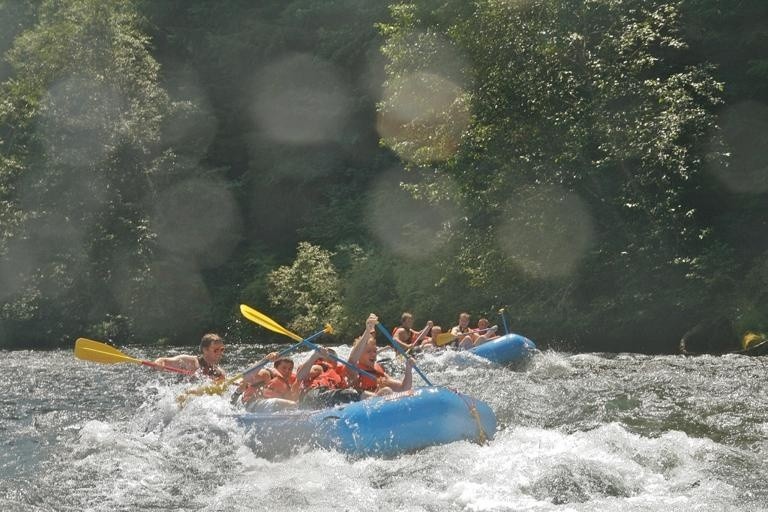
[234,385,497,463]
[412,333,536,374]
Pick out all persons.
[392,312,433,355]
[153,332,226,381]
[472,318,497,341]
[242,352,298,413]
[342,312,416,399]
[421,325,443,349]
[449,312,487,350]
[290,346,360,410]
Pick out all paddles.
[176,323,334,410]
[436,328,489,346]
[393,325,430,365]
[240,304,381,384]
[75,337,240,386]
